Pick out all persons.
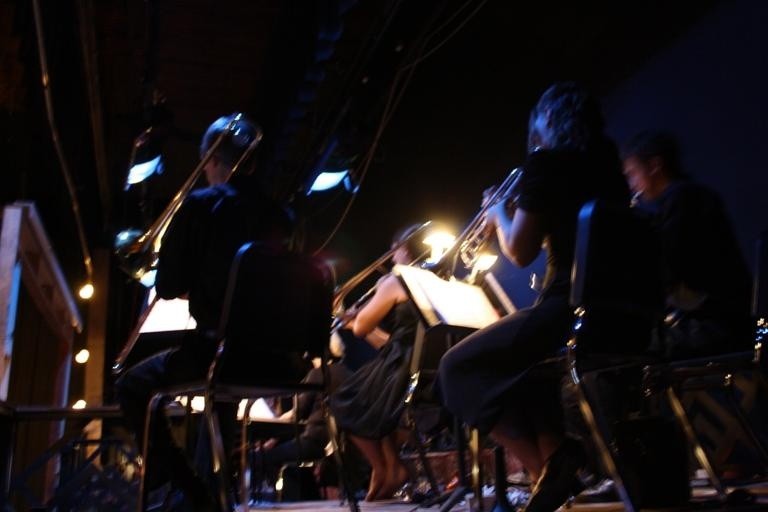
[114,81,752,511]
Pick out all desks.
[5,405,122,512]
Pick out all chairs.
[337,324,467,506]
[643,230,768,463]
[471,199,726,512]
[137,241,357,511]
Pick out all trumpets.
[422,147,543,280]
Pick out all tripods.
[414,333,479,511]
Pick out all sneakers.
[520,439,609,512]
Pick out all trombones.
[112,114,262,375]
[331,219,445,335]
